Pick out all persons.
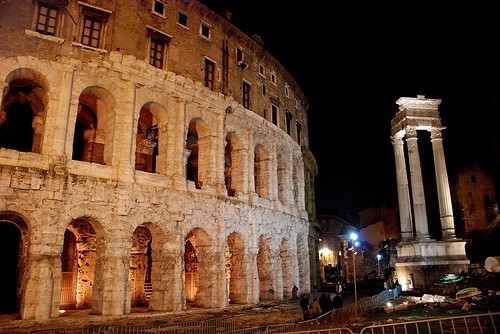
[326,293,333,322]
[333,291,343,317]
[300,294,310,321]
[309,298,322,325]
[319,294,328,322]
[292,285,298,303]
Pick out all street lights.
[350,232,358,317]
[377,254,382,277]
